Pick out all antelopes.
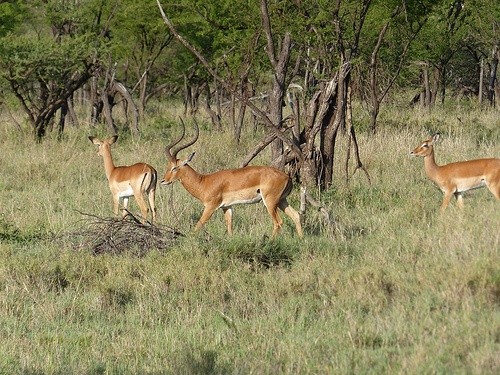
[161,116,303,236]
[409,134,500,217]
[87,135,157,222]
